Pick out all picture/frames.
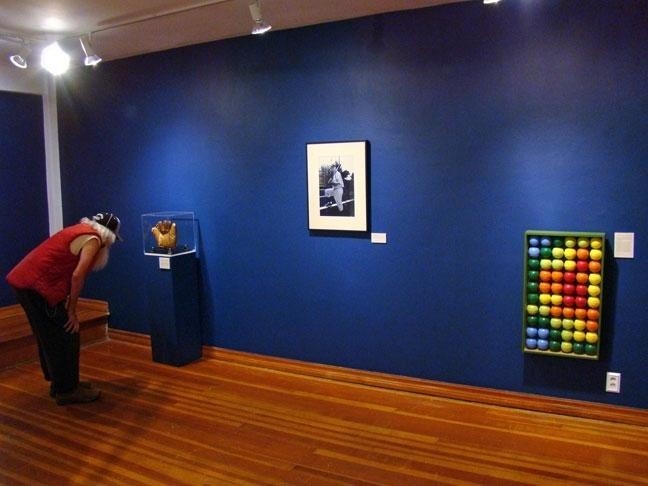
[304,140,370,238]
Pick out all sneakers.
[50,381,101,405]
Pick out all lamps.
[6,40,40,72]
[75,0,275,70]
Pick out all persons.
[5,211,125,404]
[324,164,349,215]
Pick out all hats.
[95,211,125,243]
